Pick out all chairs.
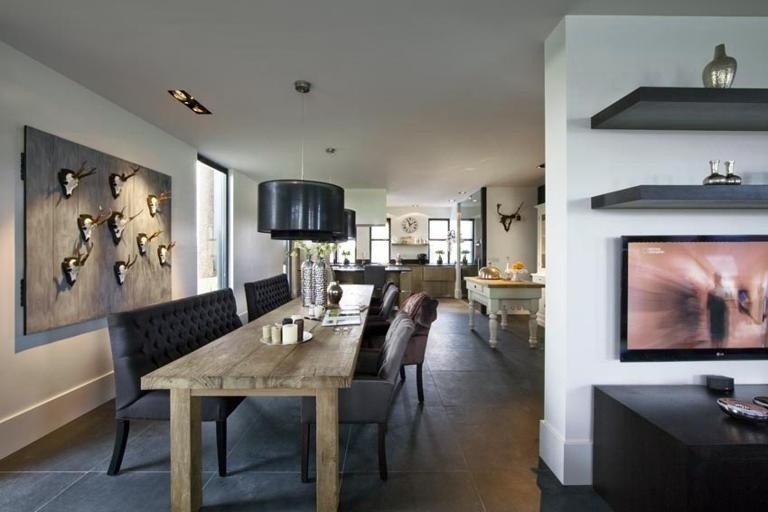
[300,309,413,484]
[358,293,437,405]
[370,284,399,318]
[373,280,395,301]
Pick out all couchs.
[244,273,293,321]
[103,288,248,478]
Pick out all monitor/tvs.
[619,234,768,363]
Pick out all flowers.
[290,238,341,256]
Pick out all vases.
[302,256,327,306]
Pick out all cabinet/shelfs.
[530,202,546,327]
[331,264,476,299]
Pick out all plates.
[258,329,313,346]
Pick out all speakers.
[707,374,735,393]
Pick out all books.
[322,304,361,325]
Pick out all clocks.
[401,216,419,234]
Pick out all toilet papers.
[282,324,297,345]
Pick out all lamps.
[312,147,357,243]
[256,81,344,235]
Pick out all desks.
[592,382,768,510]
[465,275,545,350]
[139,282,375,507]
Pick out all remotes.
[752,396,768,406]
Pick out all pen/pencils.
[304,317,323,321]
